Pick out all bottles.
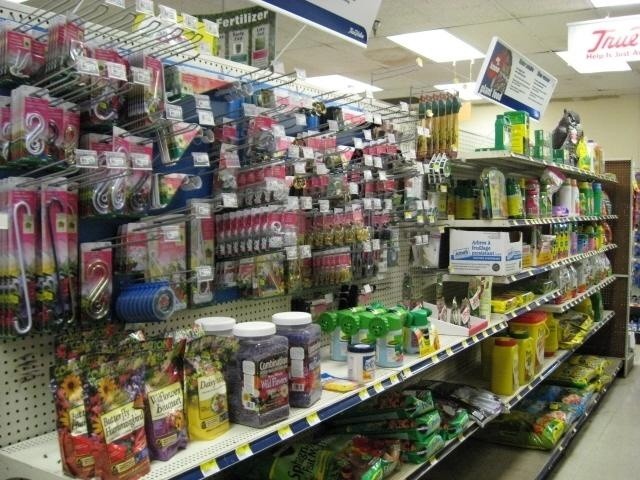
[193,311,322,429]
[480,331,536,396]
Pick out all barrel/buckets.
[495,114,513,152]
[553,179,604,217]
[508,311,551,375]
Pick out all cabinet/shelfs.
[1,150,640,480]
